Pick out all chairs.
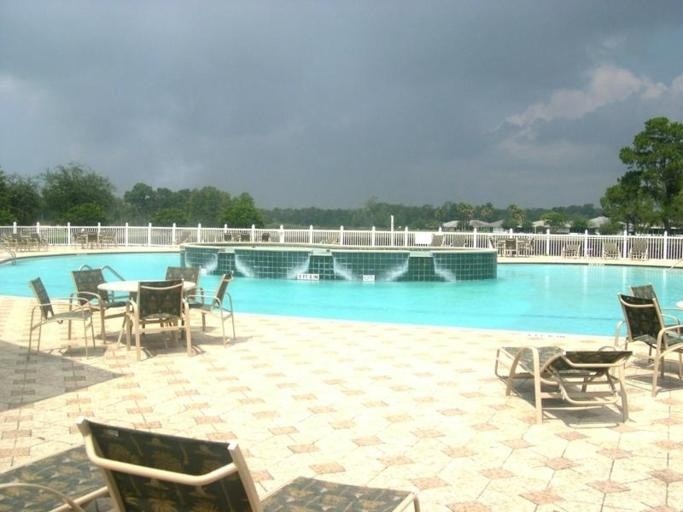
[74,418,419,512]
[0,444,111,512]
[223,231,271,243]
[614,283,683,397]
[488,235,535,258]
[26,265,236,361]
[494,340,633,426]
[561,237,649,260]
[0,228,119,253]
